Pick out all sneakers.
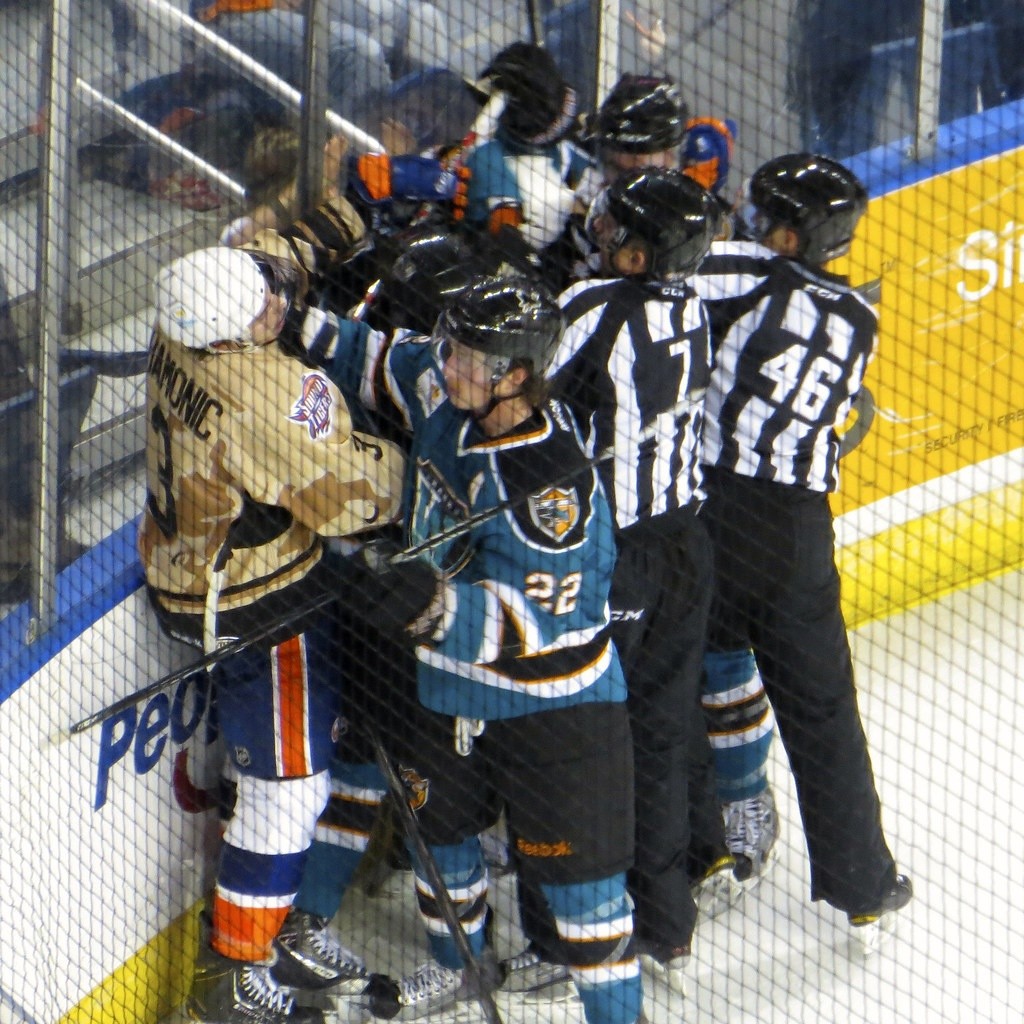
[185,942,324,1024]
[490,946,574,1004]
[388,955,495,1018]
[722,787,780,888]
[269,908,376,1022]
[847,873,911,955]
[627,928,690,996]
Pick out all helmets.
[440,274,568,379]
[384,225,488,331]
[748,154,865,265]
[153,247,266,351]
[590,73,688,155]
[606,164,719,282]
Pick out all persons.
[194,1,910,1024]
[138,247,412,1024]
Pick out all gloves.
[343,541,459,650]
[462,41,579,149]
[272,144,516,312]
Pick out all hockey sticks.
[68,448,627,737]
[341,683,506,1024]
